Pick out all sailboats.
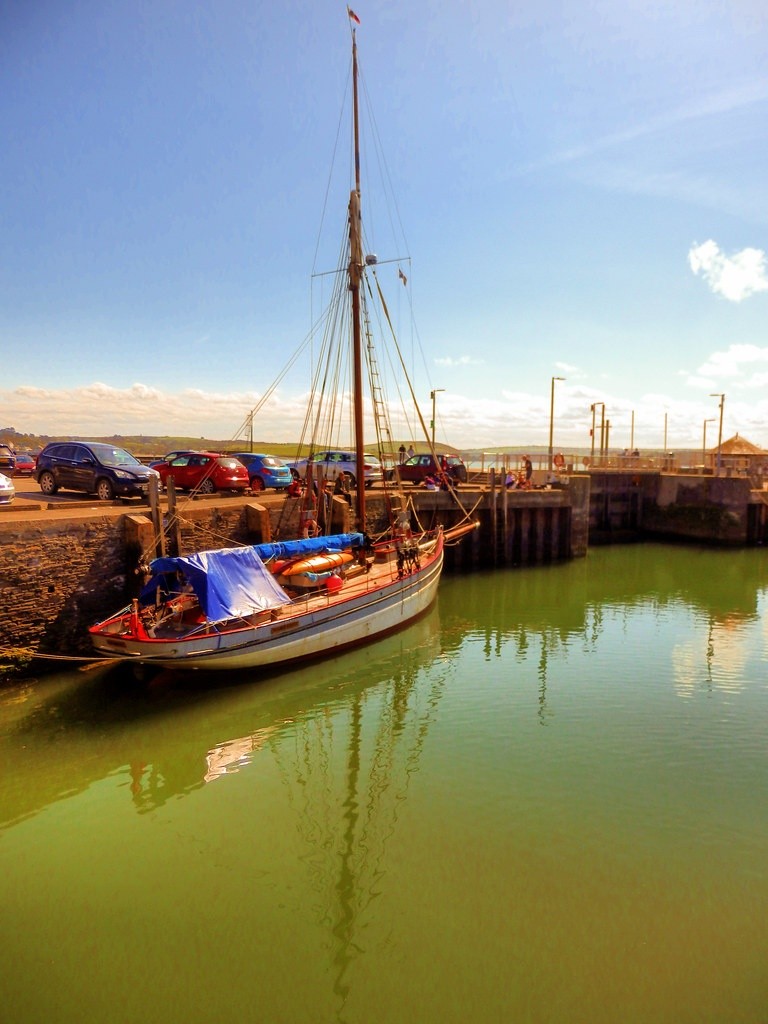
[86,2,481,671]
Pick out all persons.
[333,471,352,506]
[399,444,406,464]
[421,472,459,505]
[506,470,516,489]
[633,448,639,460]
[622,449,628,456]
[521,455,532,481]
[313,481,329,510]
[407,445,414,460]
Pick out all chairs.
[187,457,194,465]
[194,458,200,466]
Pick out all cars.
[0,444,38,504]
[147,449,295,495]
[393,453,468,489]
[285,450,383,490]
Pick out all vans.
[30,440,164,499]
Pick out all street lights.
[703,418,716,465]
[591,402,604,466]
[548,376,566,470]
[710,393,726,477]
[430,389,445,449]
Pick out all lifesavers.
[554,454,564,467]
[303,519,318,539]
